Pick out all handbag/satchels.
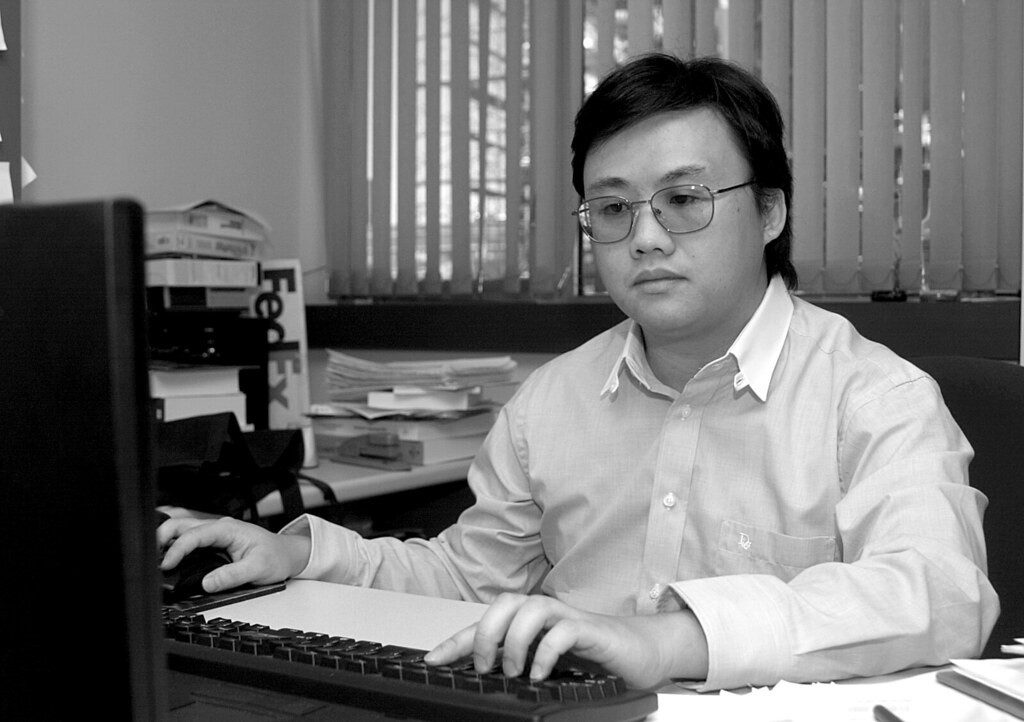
[157,409,305,519]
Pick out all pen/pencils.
[873,705,904,722]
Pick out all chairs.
[811,355,1024,656]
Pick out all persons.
[152,50,1002,693]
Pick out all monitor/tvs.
[0,197,174,722]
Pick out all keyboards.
[161,601,659,722]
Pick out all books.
[144,206,267,260]
[310,386,498,464]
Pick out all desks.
[192,578,1024,722]
[240,352,572,522]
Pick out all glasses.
[572,175,772,244]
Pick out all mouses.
[159,537,233,605]
